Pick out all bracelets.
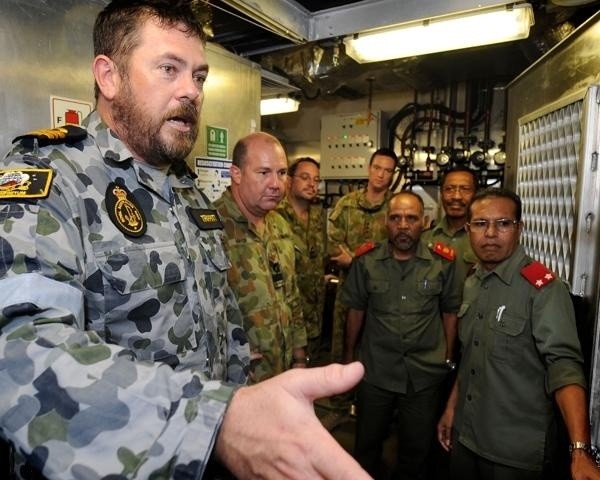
[567,441,600,463]
[292,355,312,368]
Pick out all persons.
[317,146,398,430]
[0,0,378,480]
[279,155,331,366]
[421,166,480,279]
[434,185,600,480]
[332,190,468,480]
[210,131,310,388]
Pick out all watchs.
[445,359,458,369]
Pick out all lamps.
[343,3,536,64]
[260,95,301,117]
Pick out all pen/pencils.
[495,305,507,322]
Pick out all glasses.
[441,185,472,195]
[466,218,519,232]
[293,173,320,182]
